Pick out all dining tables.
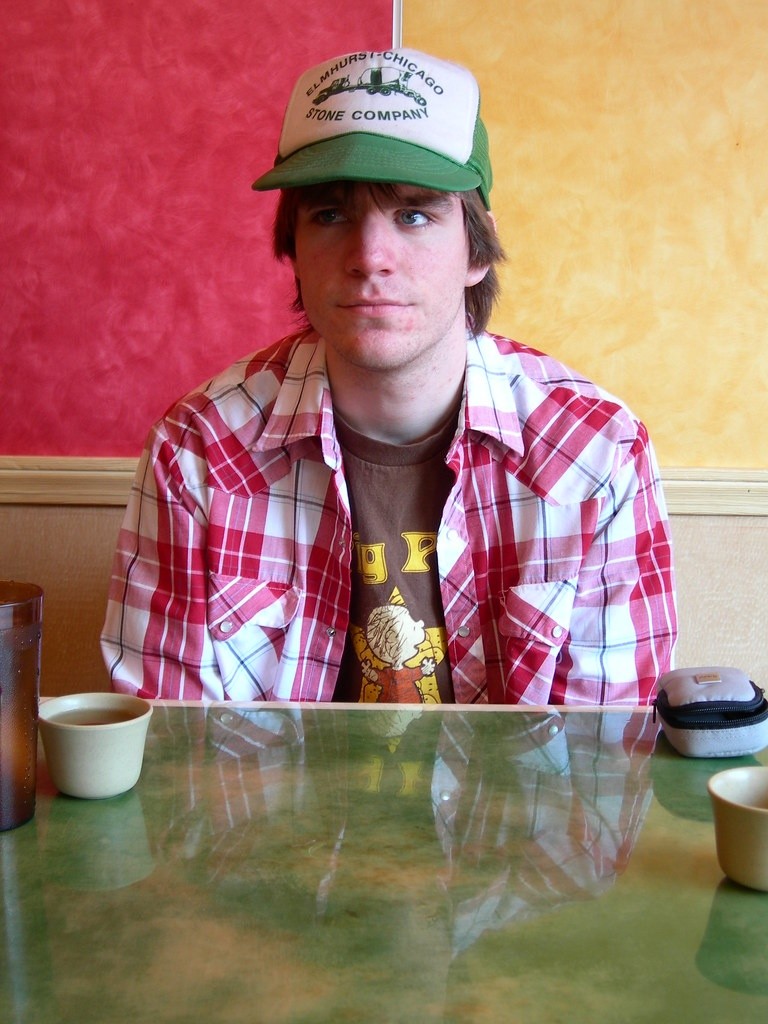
[0,696,768,1024]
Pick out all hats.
[251,48,495,211]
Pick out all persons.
[137,704,660,1023]
[101,50,677,704]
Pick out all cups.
[0,579,45,833]
[705,766,768,892]
[38,692,153,801]
